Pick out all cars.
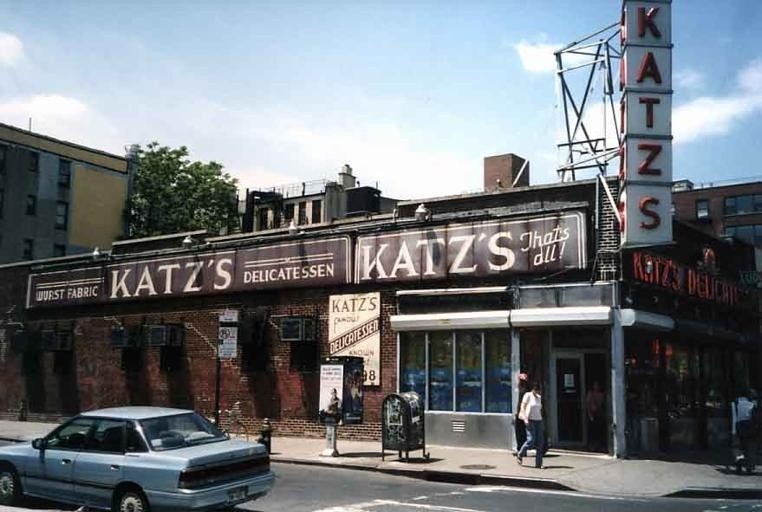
[2,405,276,512]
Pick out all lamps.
[289,219,305,238]
[182,235,199,251]
[92,247,108,262]
[415,204,432,223]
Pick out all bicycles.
[204,401,249,441]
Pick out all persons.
[514,383,529,457]
[329,388,340,412]
[730,390,757,471]
[343,371,363,413]
[584,381,609,453]
[517,383,546,468]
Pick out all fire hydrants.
[255,417,273,454]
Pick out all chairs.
[103,426,121,451]
